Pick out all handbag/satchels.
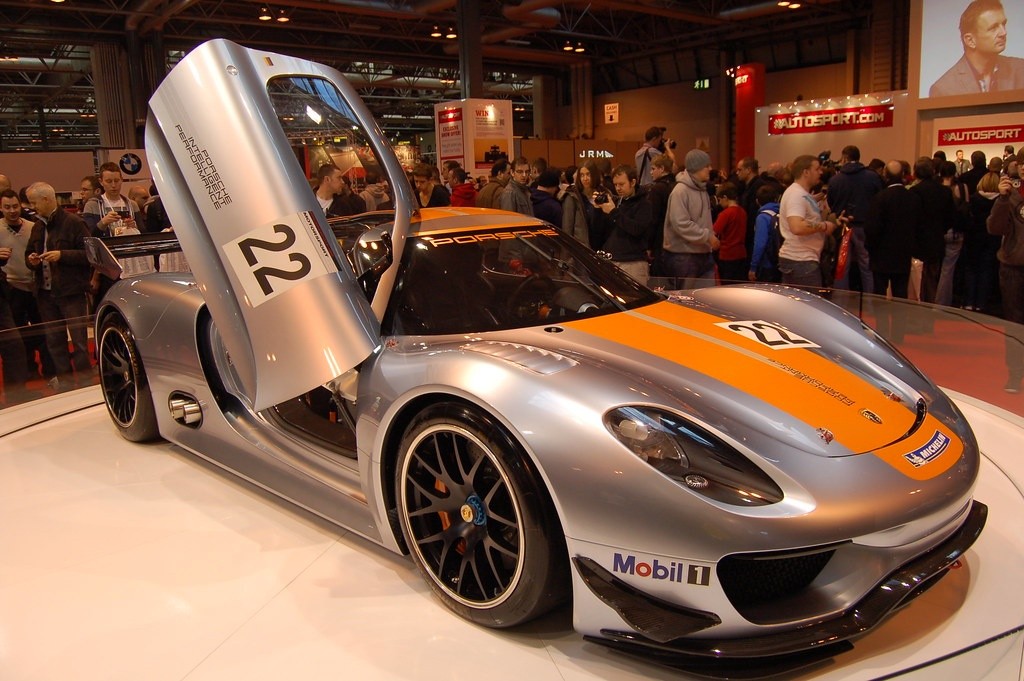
[834,221,854,279]
[886,257,923,303]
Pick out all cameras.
[35,256,45,261]
[659,139,676,150]
[594,193,608,205]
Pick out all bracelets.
[824,221,828,232]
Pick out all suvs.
[61,204,79,215]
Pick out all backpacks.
[159,226,191,272]
[101,193,154,279]
[759,208,786,250]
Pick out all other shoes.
[49,376,61,389]
[77,379,93,387]
[17,378,42,400]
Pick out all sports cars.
[87,39,989,662]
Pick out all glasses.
[80,187,94,192]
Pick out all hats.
[596,158,612,173]
[1016,147,1024,165]
[685,149,711,173]
[536,170,561,187]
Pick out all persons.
[312,127,1024,394]
[929,0,1024,97]
[0,161,195,381]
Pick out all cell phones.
[114,211,129,218]
